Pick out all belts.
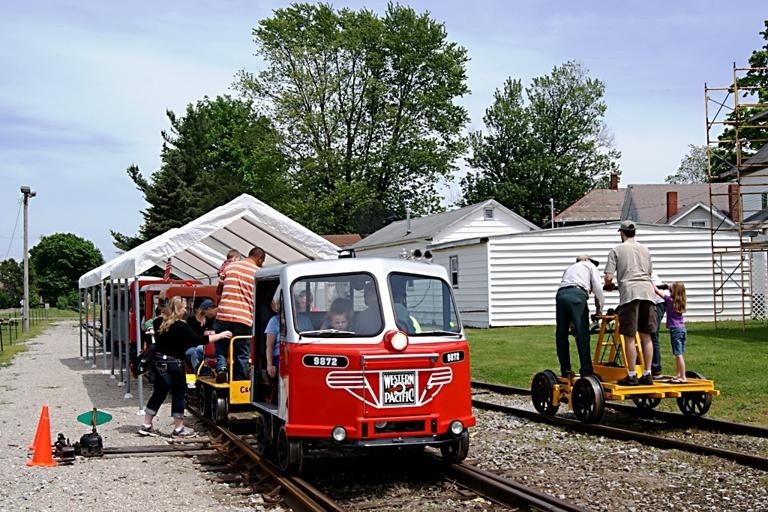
[555,285,590,294]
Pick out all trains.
[99,248,479,478]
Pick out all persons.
[637,268,669,380]
[265,282,422,409]
[603,219,658,387]
[139,247,265,439]
[650,279,687,384]
[555,253,605,380]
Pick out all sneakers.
[138,423,162,437]
[562,370,576,378]
[215,368,227,384]
[652,366,662,376]
[617,372,639,386]
[171,425,197,439]
[580,369,602,380]
[639,372,654,385]
[670,376,687,383]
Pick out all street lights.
[19,185,37,332]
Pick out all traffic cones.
[27,403,62,469]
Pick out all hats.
[575,254,600,267]
[617,220,637,232]
[199,298,215,311]
